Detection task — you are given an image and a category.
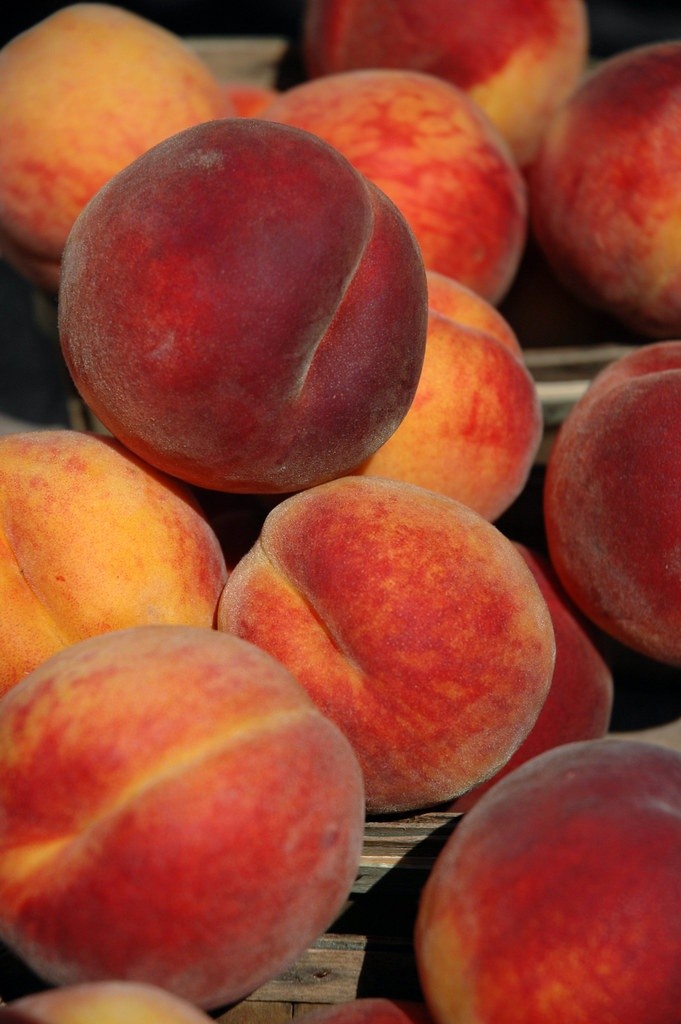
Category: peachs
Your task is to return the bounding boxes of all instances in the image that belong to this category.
[1,1,681,1024]
